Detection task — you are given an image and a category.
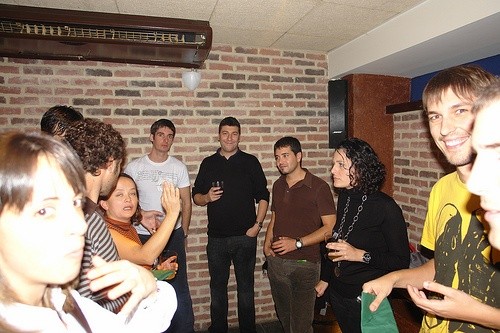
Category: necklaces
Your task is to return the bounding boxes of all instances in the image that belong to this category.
[334,194,368,267]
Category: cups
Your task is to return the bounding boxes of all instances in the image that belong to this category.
[158,250,178,280]
[212,180,223,195]
[324,230,344,261]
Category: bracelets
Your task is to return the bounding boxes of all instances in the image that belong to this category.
[183,235,189,239]
[255,220,263,226]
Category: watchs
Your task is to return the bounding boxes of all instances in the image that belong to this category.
[363,250,371,264]
[294,236,304,250]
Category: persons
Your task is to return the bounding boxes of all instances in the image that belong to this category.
[313,137,411,333]
[98,172,182,280]
[467,75,500,250]
[361,64,500,333]
[192,117,270,333]
[123,118,196,333]
[262,136,337,333]
[0,104,177,333]
